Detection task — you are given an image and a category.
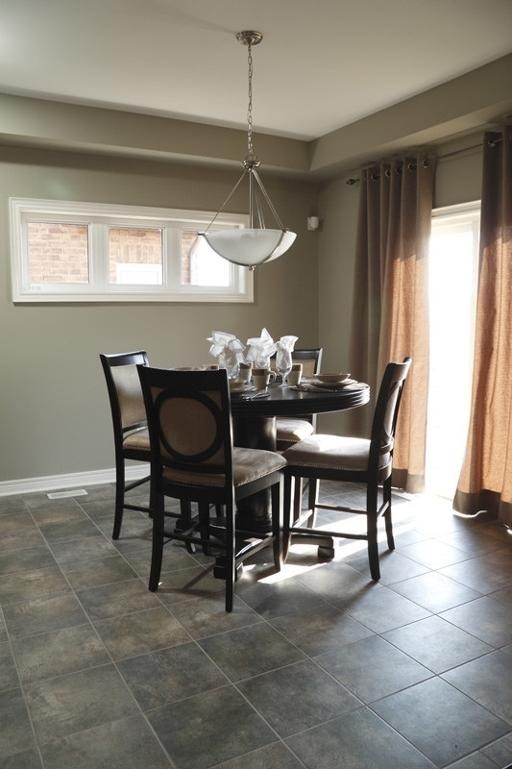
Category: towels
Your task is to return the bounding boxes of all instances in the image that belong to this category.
[273,335,299,369]
[206,330,247,366]
[246,328,279,370]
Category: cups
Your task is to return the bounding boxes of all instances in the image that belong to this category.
[240,362,252,384]
[210,365,219,370]
[252,368,277,389]
[288,363,303,386]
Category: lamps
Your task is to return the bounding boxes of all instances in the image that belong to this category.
[197,30,297,271]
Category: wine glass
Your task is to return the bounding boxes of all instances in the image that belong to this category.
[275,350,292,387]
[254,355,271,371]
[220,350,239,379]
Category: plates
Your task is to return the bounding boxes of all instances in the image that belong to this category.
[315,373,351,384]
[311,381,356,390]
